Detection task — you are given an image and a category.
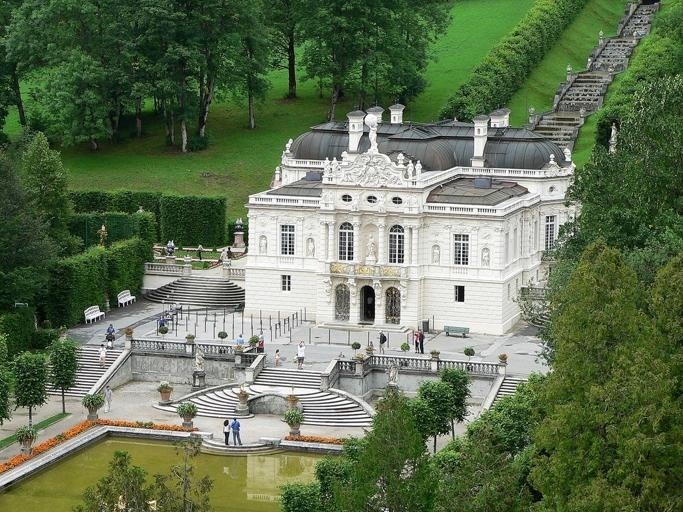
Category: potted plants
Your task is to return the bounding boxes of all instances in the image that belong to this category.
[16,425,37,448]
[499,353,508,363]
[60,325,67,337]
[430,351,440,358]
[82,394,105,420]
[248,335,259,347]
[281,408,304,436]
[185,335,195,343]
[218,331,228,354]
[464,348,475,371]
[177,402,197,428]
[158,384,173,401]
[401,343,410,366]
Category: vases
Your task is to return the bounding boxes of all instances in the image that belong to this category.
[238,393,249,404]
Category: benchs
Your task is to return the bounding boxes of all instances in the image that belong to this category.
[117,290,136,307]
[442,326,469,338]
[84,306,105,324]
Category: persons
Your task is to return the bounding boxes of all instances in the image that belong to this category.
[331,156,339,174]
[418,328,425,355]
[97,344,107,369]
[296,339,305,369]
[376,330,387,354]
[406,159,414,181]
[293,353,298,362]
[367,123,379,153]
[222,419,230,446]
[259,237,266,253]
[234,333,245,345]
[257,329,264,340]
[225,246,232,260]
[157,314,167,328]
[194,346,205,371]
[482,248,489,267]
[366,234,377,258]
[432,246,439,264]
[307,239,314,256]
[215,248,227,265]
[102,385,113,413]
[104,323,115,342]
[272,349,280,367]
[414,159,422,179]
[230,417,242,446]
[414,331,420,355]
[239,246,248,258]
[195,242,203,260]
[104,330,115,349]
[322,156,331,177]
